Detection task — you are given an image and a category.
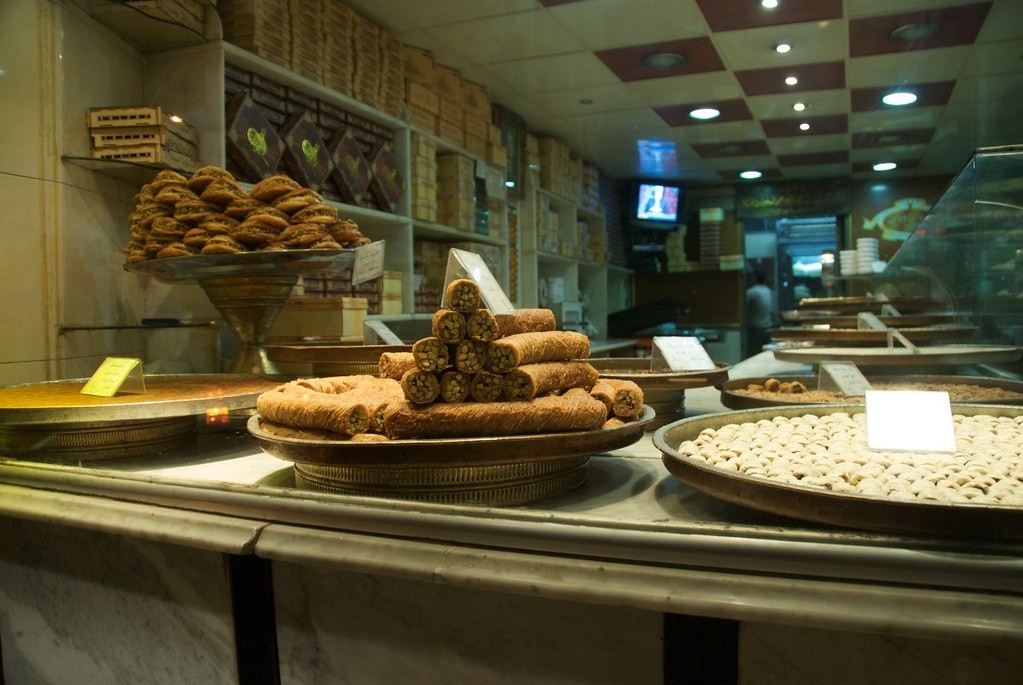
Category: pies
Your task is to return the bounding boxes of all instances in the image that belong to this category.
[124,167,371,263]
[257,279,642,443]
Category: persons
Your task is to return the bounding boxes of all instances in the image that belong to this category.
[646,186,668,214]
[744,267,773,356]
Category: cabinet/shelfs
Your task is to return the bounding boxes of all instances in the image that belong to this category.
[516,178,608,360]
[57,0,508,382]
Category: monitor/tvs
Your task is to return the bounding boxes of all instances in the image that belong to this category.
[625,177,686,231]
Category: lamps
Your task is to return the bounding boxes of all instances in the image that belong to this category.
[687,103,725,122]
[641,51,685,70]
[881,87,922,106]
[889,20,940,43]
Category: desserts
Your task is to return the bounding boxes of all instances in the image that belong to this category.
[678,379,1023,506]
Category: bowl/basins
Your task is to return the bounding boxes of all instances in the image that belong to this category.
[839,238,879,275]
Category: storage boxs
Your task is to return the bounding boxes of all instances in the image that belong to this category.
[698,208,746,273]
[83,0,608,348]
